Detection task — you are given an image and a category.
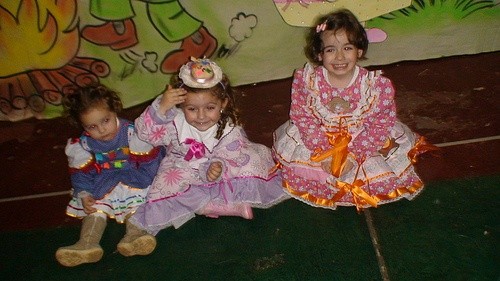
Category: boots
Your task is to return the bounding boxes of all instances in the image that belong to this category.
[117,214,156,257]
[203,204,253,219]
[55,216,107,267]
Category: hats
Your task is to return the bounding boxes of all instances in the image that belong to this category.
[180,56,222,88]
